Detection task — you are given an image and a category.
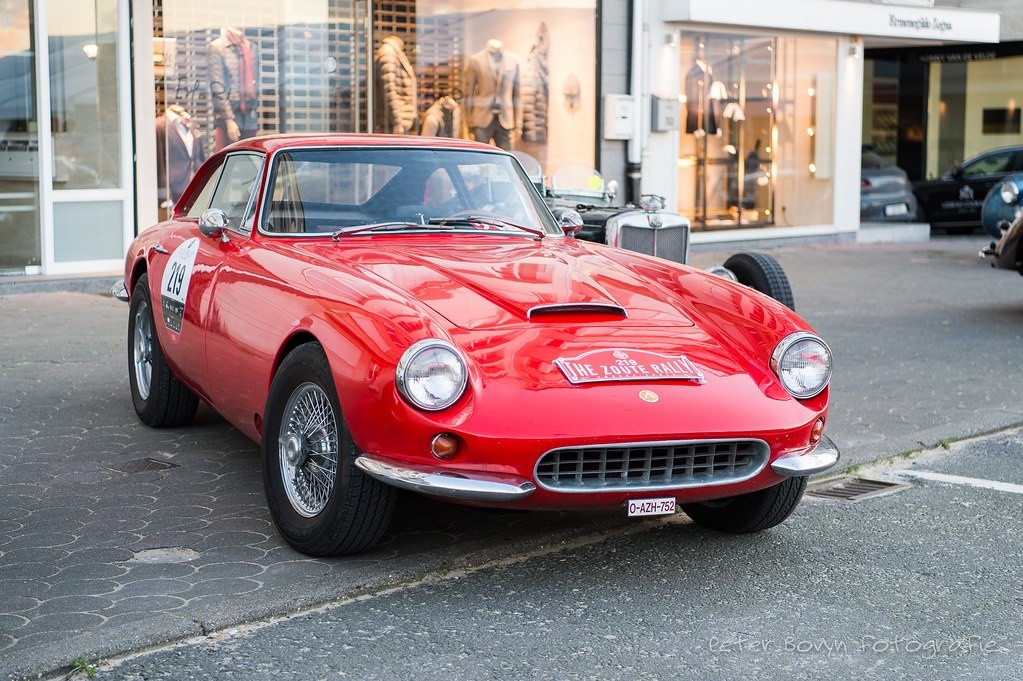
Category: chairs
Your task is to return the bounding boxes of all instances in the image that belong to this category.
[394,206,444,226]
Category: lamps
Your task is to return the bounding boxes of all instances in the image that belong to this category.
[82,44,98,59]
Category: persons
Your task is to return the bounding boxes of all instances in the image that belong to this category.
[206,25,258,153]
[155,104,205,204]
[464,39,521,152]
[520,52,548,144]
[683,59,725,136]
[419,96,458,138]
[373,33,418,135]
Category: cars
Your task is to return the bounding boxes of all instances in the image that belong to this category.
[861,145,1023,237]
[108,133,833,558]
